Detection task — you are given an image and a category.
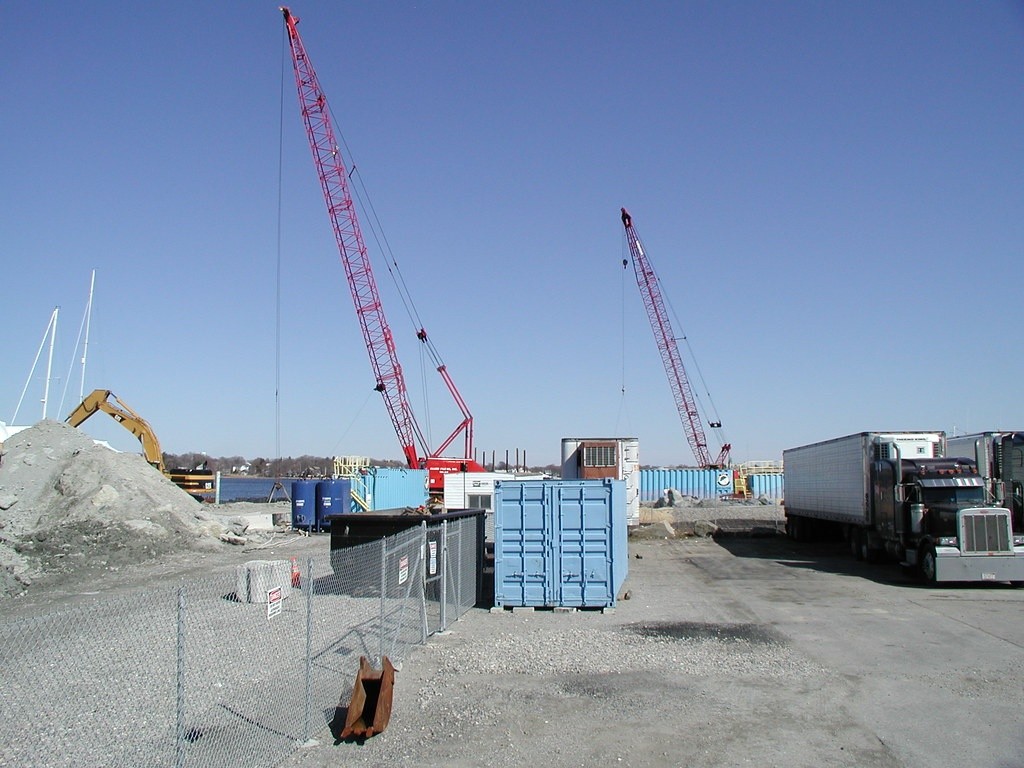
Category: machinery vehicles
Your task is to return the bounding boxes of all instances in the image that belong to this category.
[65,388,217,501]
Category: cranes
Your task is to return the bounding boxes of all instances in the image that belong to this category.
[619,204,731,472]
[276,4,488,497]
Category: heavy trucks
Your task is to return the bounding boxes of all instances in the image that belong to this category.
[781,430,993,582]
